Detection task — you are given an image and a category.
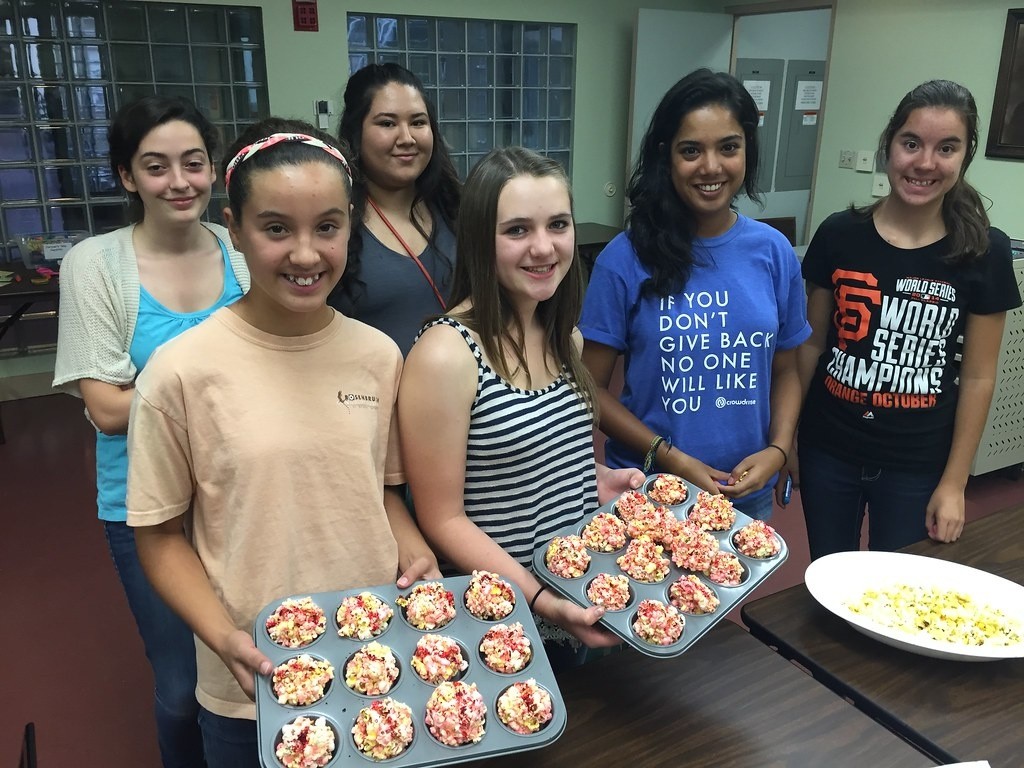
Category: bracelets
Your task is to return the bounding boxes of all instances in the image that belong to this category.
[768,444,788,467]
[644,435,673,476]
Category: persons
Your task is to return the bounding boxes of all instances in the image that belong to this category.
[398,145,645,671]
[58,93,252,768]
[125,117,446,768]
[770,79,1023,563]
[326,63,471,361]
[579,68,814,528]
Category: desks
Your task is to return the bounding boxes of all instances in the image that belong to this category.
[575,222,628,285]
[432,617,937,767]
[740,508,1024,768]
[0,262,58,336]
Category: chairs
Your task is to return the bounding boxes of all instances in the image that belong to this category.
[755,216,796,248]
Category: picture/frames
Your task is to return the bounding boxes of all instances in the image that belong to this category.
[985,8,1024,162]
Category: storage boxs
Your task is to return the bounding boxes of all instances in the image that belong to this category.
[15,232,91,271]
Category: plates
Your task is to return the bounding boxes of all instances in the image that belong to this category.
[804,551,1024,660]
